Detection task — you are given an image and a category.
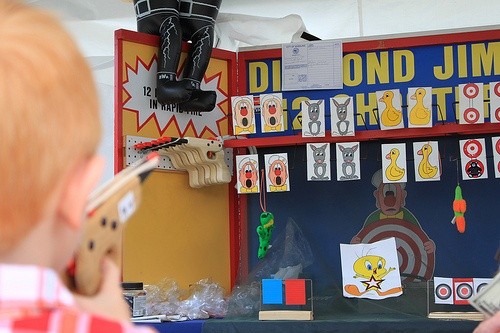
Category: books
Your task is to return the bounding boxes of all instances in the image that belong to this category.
[259,310,313,320]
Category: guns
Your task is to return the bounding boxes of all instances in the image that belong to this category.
[132,134,232,191]
[62,151,163,298]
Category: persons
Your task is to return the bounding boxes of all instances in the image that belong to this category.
[473,311,500,333]
[0,0,157,333]
